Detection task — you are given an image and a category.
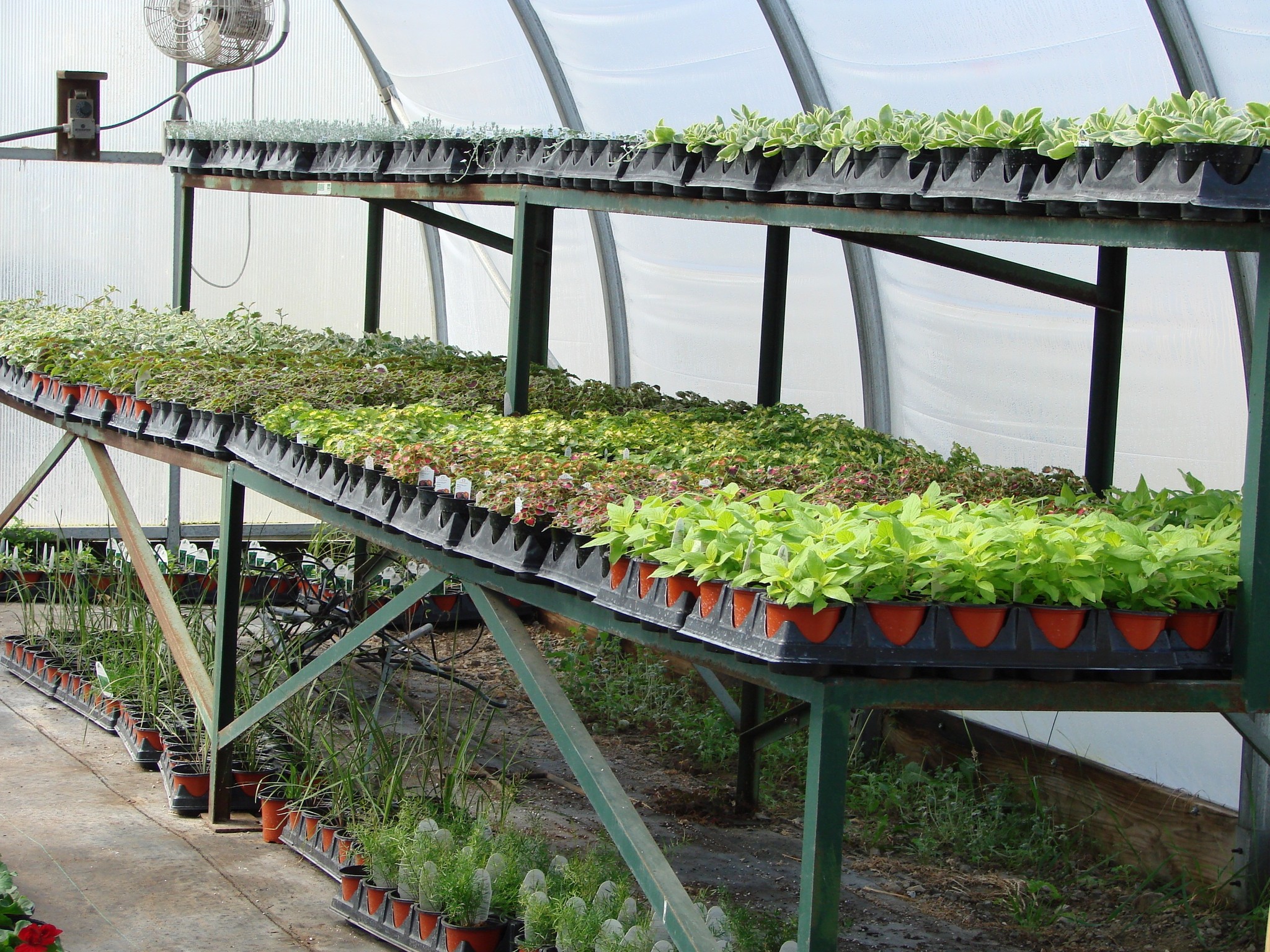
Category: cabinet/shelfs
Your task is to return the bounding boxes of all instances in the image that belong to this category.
[0,170,1270,952]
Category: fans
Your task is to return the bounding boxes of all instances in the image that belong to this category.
[142,0,276,70]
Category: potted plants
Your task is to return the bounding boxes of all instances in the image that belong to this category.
[0,98,1270,952]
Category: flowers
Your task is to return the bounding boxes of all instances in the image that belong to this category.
[0,861,65,952]
